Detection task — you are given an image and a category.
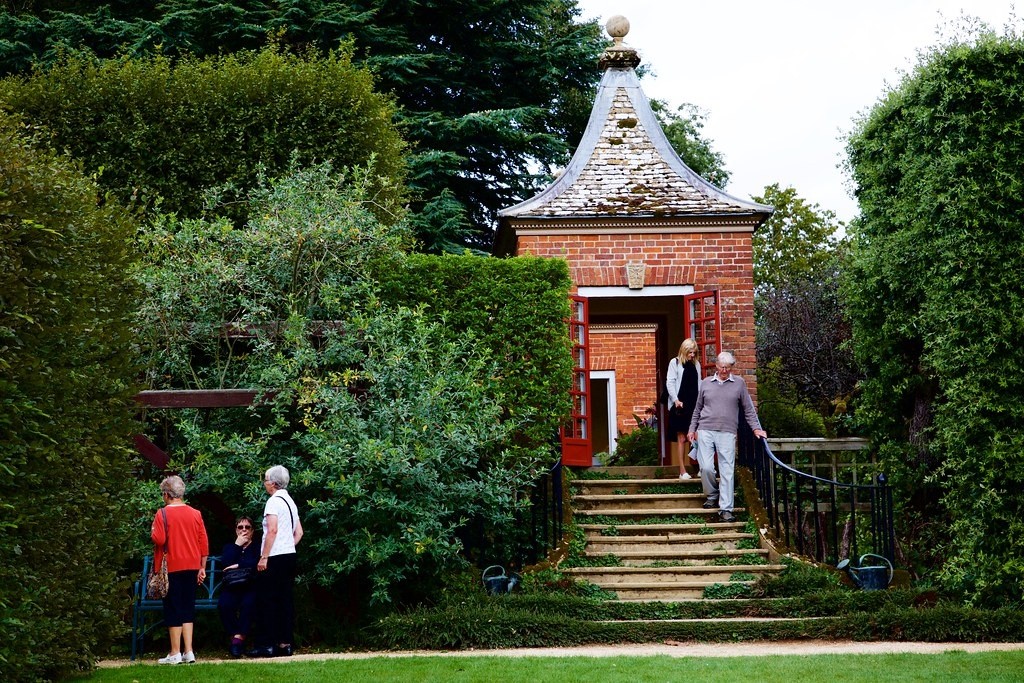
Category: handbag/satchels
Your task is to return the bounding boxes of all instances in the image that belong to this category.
[147,553,169,600]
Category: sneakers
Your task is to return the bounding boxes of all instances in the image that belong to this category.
[182,650,196,663]
[158,651,182,664]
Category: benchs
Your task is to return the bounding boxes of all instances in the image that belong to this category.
[131,556,222,660]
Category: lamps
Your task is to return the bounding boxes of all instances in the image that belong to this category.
[625,261,647,290]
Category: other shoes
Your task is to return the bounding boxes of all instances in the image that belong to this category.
[242,645,274,657]
[717,510,735,522]
[228,644,241,657]
[679,472,692,479]
[697,471,702,478]
[703,498,719,508]
[276,643,293,655]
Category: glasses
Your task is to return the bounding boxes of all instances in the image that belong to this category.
[236,525,252,530]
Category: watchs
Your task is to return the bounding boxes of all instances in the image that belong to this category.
[261,556,268,559]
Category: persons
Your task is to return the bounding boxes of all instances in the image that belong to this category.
[687,351,767,521]
[666,338,702,480]
[150,475,209,665]
[218,515,260,656]
[253,465,303,657]
